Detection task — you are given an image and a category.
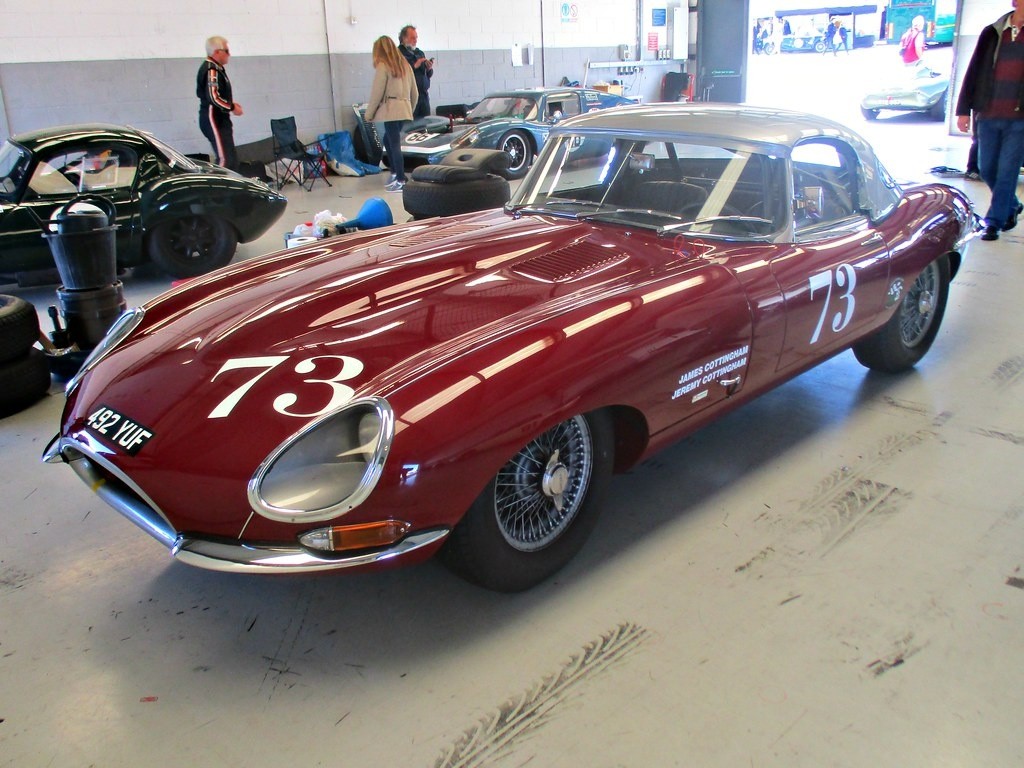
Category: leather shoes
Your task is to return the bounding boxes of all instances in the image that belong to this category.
[981,225,1000,241]
[1001,200,1023,231]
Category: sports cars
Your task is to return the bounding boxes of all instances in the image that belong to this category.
[860,62,951,121]
[377,86,654,182]
[39,101,989,595]
[0,123,288,279]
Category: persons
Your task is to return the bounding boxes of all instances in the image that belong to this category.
[196,36,243,174]
[821,18,838,56]
[398,26,433,117]
[365,36,419,192]
[835,23,849,55]
[964,109,984,181]
[752,18,791,55]
[900,15,924,67]
[955,0,1024,240]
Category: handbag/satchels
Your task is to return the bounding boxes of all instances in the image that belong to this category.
[899,48,906,55]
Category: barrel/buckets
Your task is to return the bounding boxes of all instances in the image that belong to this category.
[41,224,119,290]
[55,279,127,350]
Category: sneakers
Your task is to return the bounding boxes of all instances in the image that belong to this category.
[384,174,398,188]
[386,181,405,193]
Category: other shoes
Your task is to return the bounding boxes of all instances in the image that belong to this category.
[965,172,984,181]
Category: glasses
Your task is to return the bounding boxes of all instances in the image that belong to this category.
[218,49,229,54]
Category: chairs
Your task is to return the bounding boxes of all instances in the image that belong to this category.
[271,116,333,191]
[631,180,707,215]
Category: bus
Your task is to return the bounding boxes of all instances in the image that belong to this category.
[755,3,878,55]
[883,0,958,46]
